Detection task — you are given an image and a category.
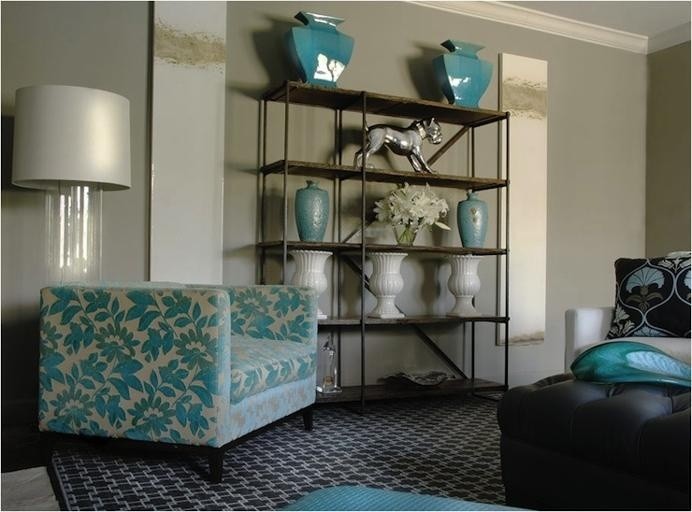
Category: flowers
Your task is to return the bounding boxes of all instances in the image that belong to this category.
[371,181,451,244]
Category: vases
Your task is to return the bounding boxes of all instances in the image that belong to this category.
[363,252,404,318]
[294,178,329,244]
[456,191,489,249]
[433,39,495,108]
[288,250,333,318]
[388,216,422,247]
[286,11,356,87]
[443,253,484,318]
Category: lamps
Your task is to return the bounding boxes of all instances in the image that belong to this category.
[11,85,131,282]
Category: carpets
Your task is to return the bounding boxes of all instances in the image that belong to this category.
[49,388,510,511]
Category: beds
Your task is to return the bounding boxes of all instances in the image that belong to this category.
[496,250,690,511]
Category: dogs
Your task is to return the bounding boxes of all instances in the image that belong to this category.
[354,115,443,174]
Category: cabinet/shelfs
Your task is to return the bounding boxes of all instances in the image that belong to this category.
[259,80,511,419]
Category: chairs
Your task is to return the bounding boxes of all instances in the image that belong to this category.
[34,282,318,486]
